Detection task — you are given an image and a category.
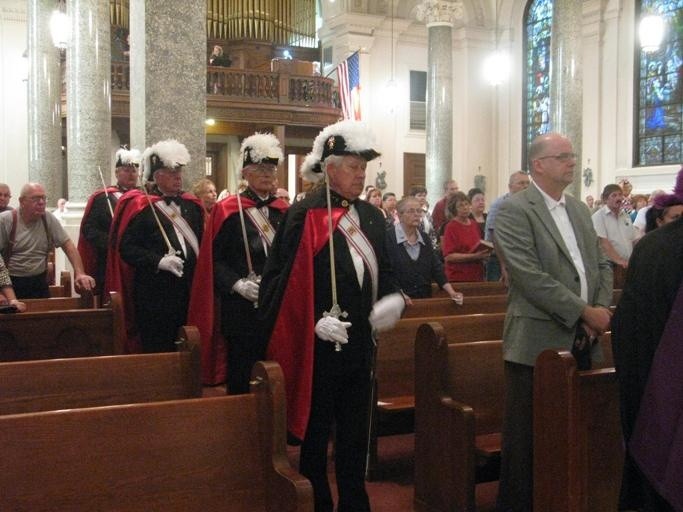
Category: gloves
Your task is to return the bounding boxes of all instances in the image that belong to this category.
[232,279,260,302]
[368,293,406,332]
[158,256,185,278]
[315,316,352,345]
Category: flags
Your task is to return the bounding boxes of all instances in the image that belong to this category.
[334,51,365,119]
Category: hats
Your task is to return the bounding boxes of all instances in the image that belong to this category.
[299,119,383,184]
[142,139,191,181]
[115,144,142,167]
[240,130,285,179]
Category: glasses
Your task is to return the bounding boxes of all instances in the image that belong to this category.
[539,152,579,160]
[22,194,47,201]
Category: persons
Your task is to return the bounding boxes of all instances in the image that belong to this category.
[0,186,12,212]
[0,183,96,313]
[123,35,130,62]
[207,45,223,93]
[312,60,322,77]
[489,135,616,511]
[615,217,683,508]
[75,150,142,295]
[185,133,291,397]
[51,197,68,215]
[116,139,205,353]
[192,168,682,308]
[247,119,410,509]
